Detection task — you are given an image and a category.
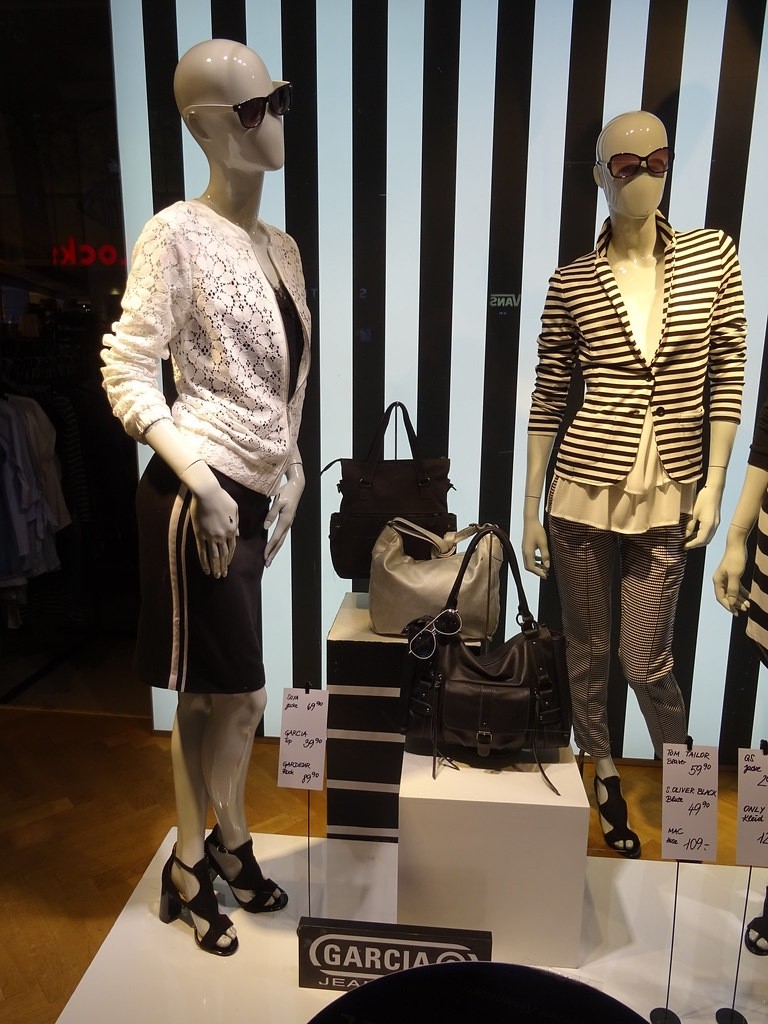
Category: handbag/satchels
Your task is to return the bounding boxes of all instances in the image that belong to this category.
[369,517,503,641]
[400,526,573,797]
[320,401,457,578]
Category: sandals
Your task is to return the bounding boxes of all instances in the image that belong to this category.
[594,771,642,859]
[744,887,768,956]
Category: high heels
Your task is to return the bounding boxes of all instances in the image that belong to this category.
[159,842,238,957]
[205,823,288,912]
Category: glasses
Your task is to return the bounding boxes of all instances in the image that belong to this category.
[409,609,462,661]
[595,147,675,179]
[184,80,292,128]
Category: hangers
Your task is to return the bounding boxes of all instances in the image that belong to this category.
[0,355,60,399]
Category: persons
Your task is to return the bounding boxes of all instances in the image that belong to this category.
[101,37,311,957]
[713,314,768,959]
[521,111,747,861]
